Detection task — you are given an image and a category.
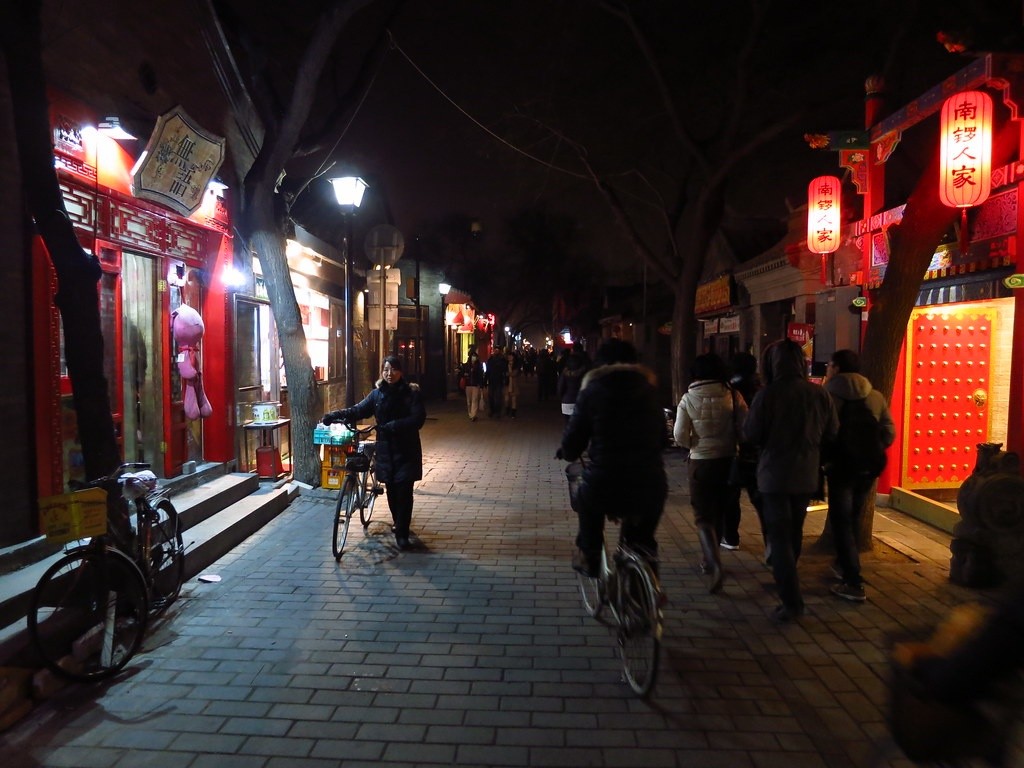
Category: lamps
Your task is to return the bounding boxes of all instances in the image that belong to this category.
[94,115,138,140]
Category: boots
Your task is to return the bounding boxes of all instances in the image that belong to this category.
[505,406,511,415]
[697,526,725,594]
[512,408,517,418]
[698,529,722,574]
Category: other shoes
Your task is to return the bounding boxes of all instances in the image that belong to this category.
[391,523,396,533]
[396,536,411,551]
[761,580,779,594]
[774,604,804,622]
[571,556,601,577]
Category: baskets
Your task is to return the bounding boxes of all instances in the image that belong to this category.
[313,427,355,445]
[331,435,379,473]
[565,460,591,513]
[38,484,108,546]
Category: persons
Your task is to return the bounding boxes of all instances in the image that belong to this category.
[674,353,749,595]
[720,352,772,565]
[556,337,676,606]
[742,338,840,622]
[459,342,592,422]
[322,358,427,549]
[822,349,894,601]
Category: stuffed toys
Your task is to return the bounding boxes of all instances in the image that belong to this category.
[170,303,213,421]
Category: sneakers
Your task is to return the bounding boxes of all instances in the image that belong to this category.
[720,537,739,550]
[832,584,866,601]
[829,559,844,580]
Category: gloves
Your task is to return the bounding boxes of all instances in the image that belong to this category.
[381,420,400,435]
[323,413,337,426]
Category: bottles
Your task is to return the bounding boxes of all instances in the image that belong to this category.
[327,446,353,478]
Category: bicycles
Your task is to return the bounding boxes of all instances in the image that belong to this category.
[322,417,390,559]
[552,451,666,704]
[28,462,196,688]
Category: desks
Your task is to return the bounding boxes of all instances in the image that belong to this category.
[243,418,293,482]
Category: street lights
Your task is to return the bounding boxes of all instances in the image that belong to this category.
[326,174,371,452]
[437,278,451,401]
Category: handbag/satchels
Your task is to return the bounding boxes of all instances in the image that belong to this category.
[479,391,486,412]
[457,376,466,389]
[732,390,753,489]
[501,370,509,385]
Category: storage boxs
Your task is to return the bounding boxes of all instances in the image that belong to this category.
[313,427,354,446]
[320,442,353,468]
[322,468,349,489]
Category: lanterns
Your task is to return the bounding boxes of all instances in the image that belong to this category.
[939,91,992,251]
[807,175,841,282]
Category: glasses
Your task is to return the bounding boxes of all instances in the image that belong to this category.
[824,361,832,366]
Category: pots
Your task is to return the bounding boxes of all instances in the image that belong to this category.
[250,401,282,424]
